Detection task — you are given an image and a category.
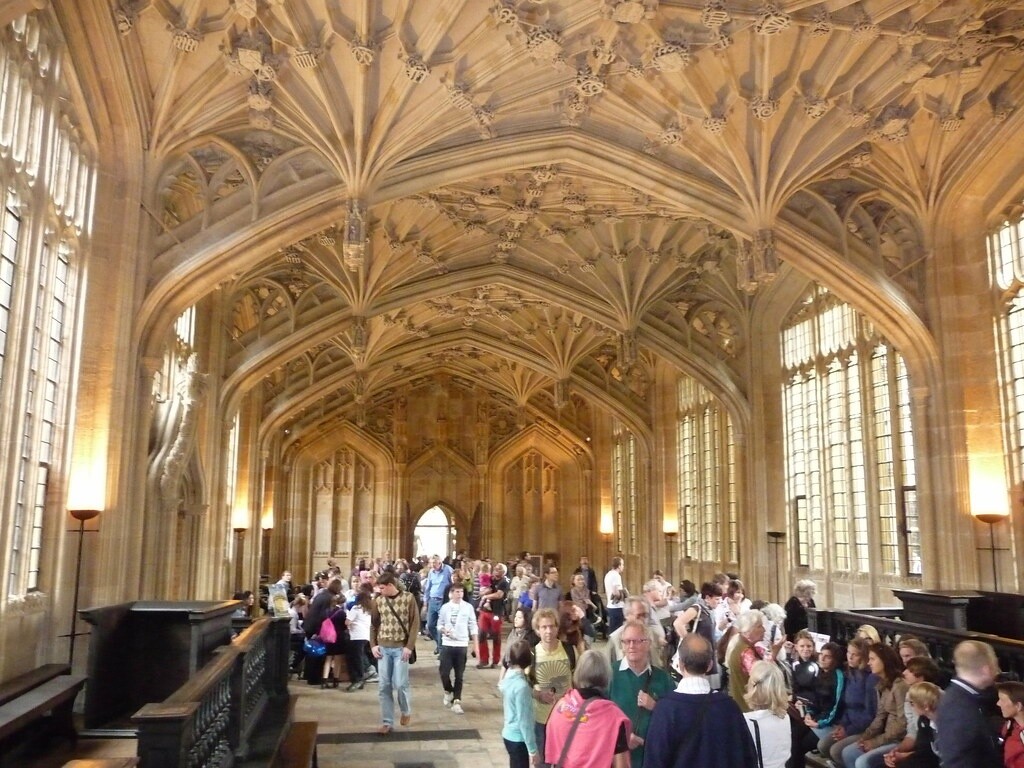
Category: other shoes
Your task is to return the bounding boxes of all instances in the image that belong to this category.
[382,724,392,735]
[425,636,432,641]
[400,714,410,724]
[483,603,493,612]
[477,607,483,612]
[365,672,379,682]
[491,663,497,669]
[476,661,489,668]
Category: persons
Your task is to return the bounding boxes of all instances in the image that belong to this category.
[642,634,758,768]
[608,620,677,768]
[742,661,806,768]
[370,574,420,734]
[523,607,579,768]
[785,579,1024,768]
[436,583,480,714]
[545,651,631,768]
[230,550,786,711]
[497,640,541,768]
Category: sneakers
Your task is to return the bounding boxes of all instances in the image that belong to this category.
[443,691,453,705]
[451,703,464,714]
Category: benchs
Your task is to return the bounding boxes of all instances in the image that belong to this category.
[0,675,89,768]
[0,664,75,725]
[267,722,318,768]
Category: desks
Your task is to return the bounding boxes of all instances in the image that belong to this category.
[77,599,242,733]
[895,589,991,633]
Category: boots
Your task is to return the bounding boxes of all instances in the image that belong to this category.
[333,678,339,687]
[321,678,331,688]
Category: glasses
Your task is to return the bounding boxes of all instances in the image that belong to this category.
[621,638,647,645]
[629,612,649,618]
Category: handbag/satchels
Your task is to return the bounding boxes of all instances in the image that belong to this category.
[404,634,417,664]
[321,619,337,643]
